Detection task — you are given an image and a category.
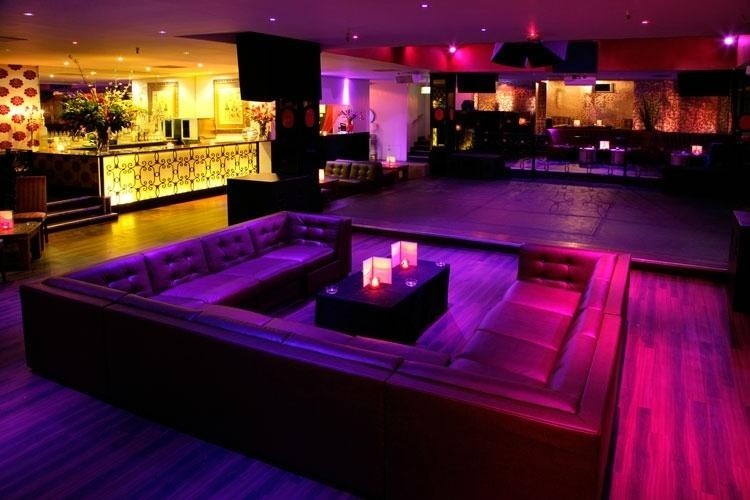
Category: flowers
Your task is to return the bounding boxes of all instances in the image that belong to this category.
[247,103,275,123]
[54,54,153,144]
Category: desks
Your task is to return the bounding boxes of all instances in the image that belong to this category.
[314,250,454,346]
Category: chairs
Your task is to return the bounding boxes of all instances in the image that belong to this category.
[12,175,49,250]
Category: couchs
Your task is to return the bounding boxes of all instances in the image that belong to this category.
[324,159,394,193]
[548,125,648,165]
[23,210,631,500]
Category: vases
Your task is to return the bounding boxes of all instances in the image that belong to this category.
[95,126,108,153]
[259,122,267,139]
